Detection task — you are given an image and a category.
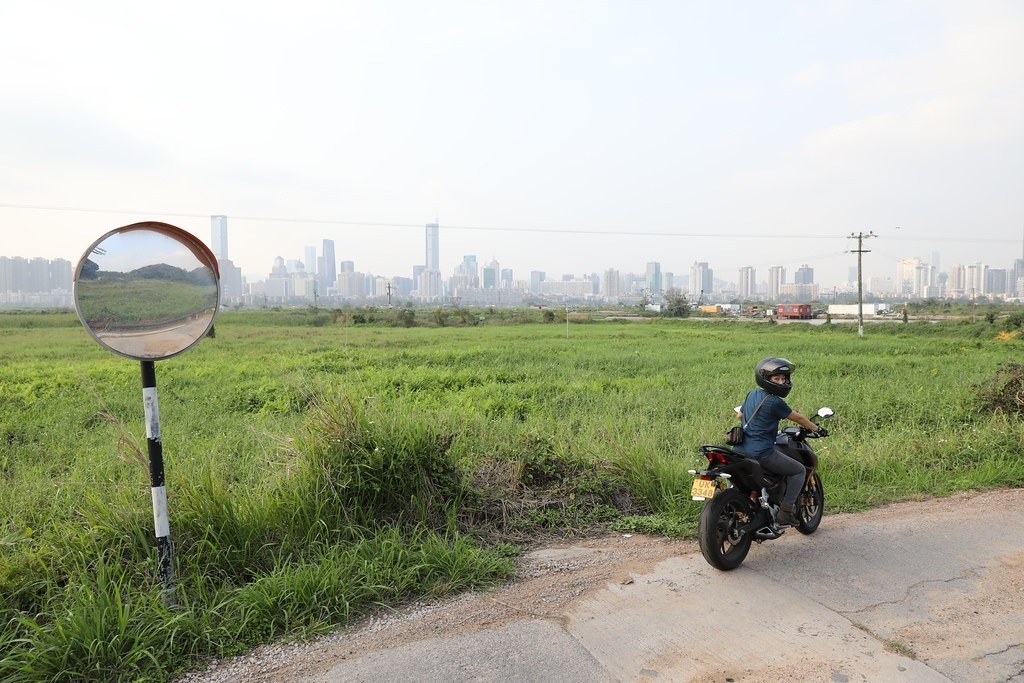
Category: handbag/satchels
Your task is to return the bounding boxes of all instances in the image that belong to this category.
[726,427,744,445]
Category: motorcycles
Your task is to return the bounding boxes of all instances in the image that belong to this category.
[690,407,835,572]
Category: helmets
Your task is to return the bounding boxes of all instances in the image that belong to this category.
[755,357,793,398]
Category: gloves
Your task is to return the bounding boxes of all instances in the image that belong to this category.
[813,426,827,437]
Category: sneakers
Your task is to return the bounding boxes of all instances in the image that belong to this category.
[777,510,800,526]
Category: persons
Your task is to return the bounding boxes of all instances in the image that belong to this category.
[731,356,828,527]
